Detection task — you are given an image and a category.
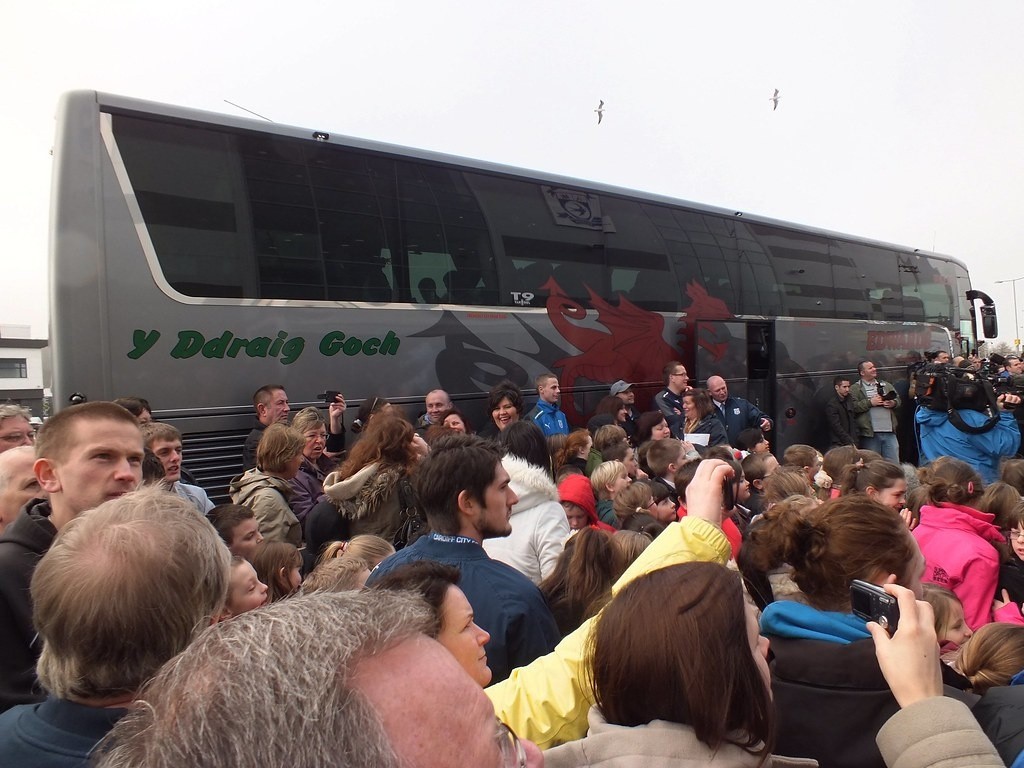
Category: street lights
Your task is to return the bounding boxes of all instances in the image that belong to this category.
[995,276,1024,355]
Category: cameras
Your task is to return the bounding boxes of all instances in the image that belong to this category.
[876,387,898,408]
[720,475,735,510]
[849,578,900,634]
[317,390,341,402]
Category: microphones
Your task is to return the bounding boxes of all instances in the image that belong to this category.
[1006,373,1023,387]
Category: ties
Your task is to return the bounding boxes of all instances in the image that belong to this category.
[720,404,725,419]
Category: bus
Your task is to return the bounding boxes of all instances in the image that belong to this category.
[43,86,998,505]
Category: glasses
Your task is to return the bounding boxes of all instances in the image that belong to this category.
[496,717,527,768]
[667,372,688,379]
[645,498,658,509]
[1004,531,1024,540]
[303,431,330,442]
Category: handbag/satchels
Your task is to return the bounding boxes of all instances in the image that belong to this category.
[393,476,427,552]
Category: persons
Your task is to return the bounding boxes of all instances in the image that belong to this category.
[0,349,1024,768]
[418,276,439,303]
[443,262,736,313]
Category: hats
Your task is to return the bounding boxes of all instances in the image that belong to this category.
[959,360,974,369]
[610,380,636,397]
